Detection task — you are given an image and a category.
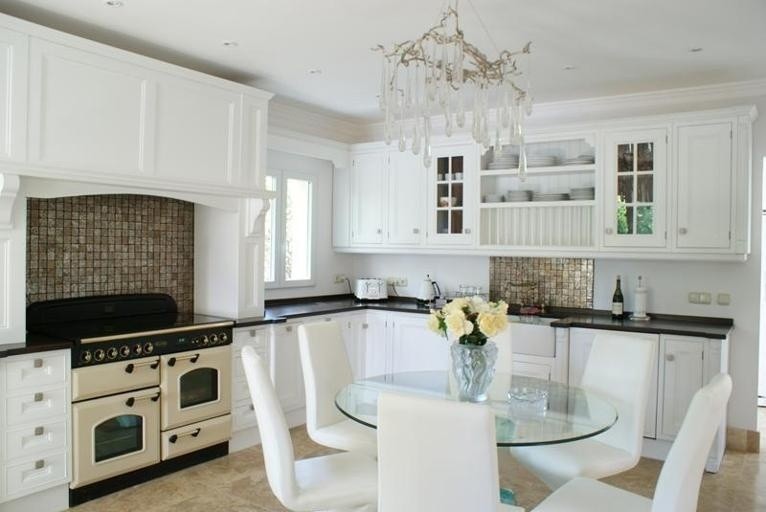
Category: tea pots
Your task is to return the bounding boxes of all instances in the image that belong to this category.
[416,274,442,304]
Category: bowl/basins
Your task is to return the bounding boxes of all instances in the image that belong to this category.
[439,197,458,208]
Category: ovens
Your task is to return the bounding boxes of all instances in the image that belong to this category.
[70,345,233,506]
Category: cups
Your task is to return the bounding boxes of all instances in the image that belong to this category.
[453,284,482,298]
[437,171,463,180]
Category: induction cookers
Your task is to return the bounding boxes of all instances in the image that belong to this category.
[33,311,234,368]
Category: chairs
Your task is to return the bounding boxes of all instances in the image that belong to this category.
[529,368,737,511]
[238,342,379,511]
[509,328,664,493]
[369,390,526,512]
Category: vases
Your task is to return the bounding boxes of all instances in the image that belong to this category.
[447,339,500,400]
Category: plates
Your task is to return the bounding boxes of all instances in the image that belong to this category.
[507,386,548,403]
[488,152,594,169]
[484,187,594,201]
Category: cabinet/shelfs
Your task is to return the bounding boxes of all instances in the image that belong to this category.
[671,104,760,263]
[330,133,432,253]
[568,312,662,460]
[480,122,600,257]
[229,296,446,454]
[0,343,71,511]
[430,132,485,256]
[601,110,671,260]
[653,316,738,474]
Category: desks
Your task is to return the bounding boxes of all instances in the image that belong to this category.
[332,363,619,507]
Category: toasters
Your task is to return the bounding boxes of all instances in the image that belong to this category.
[353,277,390,303]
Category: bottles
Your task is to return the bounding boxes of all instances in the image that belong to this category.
[611,275,623,321]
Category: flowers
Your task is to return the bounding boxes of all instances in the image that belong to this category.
[426,295,511,347]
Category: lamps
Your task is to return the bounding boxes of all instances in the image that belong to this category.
[369,0,542,185]
